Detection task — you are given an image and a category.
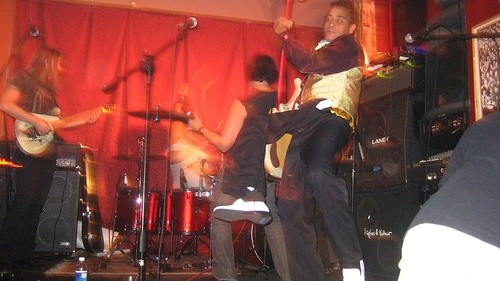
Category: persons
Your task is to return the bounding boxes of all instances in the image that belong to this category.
[0,45,102,269]
[398,110,500,281]
[186,54,291,281]
[165,124,214,187]
[212,0,365,281]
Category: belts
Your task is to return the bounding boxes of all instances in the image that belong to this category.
[329,107,355,133]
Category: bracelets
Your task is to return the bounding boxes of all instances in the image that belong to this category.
[198,125,205,134]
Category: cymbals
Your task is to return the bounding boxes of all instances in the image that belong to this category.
[113,151,167,163]
[126,108,192,123]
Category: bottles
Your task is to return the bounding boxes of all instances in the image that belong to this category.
[75,257,87,281]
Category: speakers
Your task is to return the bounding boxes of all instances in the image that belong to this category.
[348,185,425,281]
[425,0,471,116]
[350,91,424,190]
[31,141,81,252]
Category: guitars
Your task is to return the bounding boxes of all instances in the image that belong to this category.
[264,77,302,179]
[14,102,120,156]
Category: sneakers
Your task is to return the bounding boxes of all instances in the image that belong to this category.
[342,258,365,281]
[213,198,272,225]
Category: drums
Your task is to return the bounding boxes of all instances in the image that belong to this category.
[113,187,165,234]
[231,219,272,268]
[161,188,212,236]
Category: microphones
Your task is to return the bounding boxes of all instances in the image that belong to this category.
[405,23,439,43]
[28,24,39,37]
[176,17,197,29]
